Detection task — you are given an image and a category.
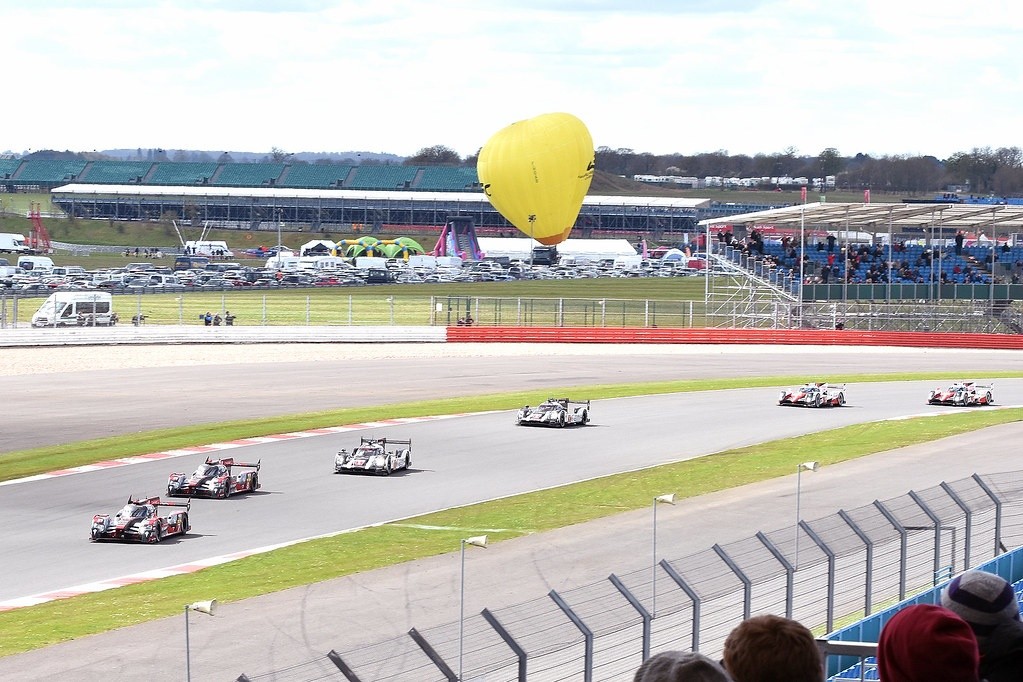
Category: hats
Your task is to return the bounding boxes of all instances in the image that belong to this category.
[633,650,734,682]
[940,569,1020,637]
[876,603,980,682]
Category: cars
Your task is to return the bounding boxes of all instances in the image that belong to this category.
[0,245,709,297]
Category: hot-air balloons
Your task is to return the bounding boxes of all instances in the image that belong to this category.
[476,112,596,268]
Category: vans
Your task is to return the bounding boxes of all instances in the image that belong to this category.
[31,291,112,329]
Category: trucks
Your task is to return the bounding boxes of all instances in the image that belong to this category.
[0,232,30,254]
[185,240,234,261]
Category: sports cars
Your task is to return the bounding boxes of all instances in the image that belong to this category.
[776,382,846,408]
[517,398,591,428]
[926,381,994,407]
[88,495,193,543]
[335,436,411,476]
[167,457,264,499]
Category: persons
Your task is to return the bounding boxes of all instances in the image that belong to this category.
[124,246,161,259]
[77,313,146,326]
[835,322,845,330]
[877,571,1023,682]
[715,230,1019,285]
[183,245,229,258]
[633,615,825,682]
[205,311,236,326]
